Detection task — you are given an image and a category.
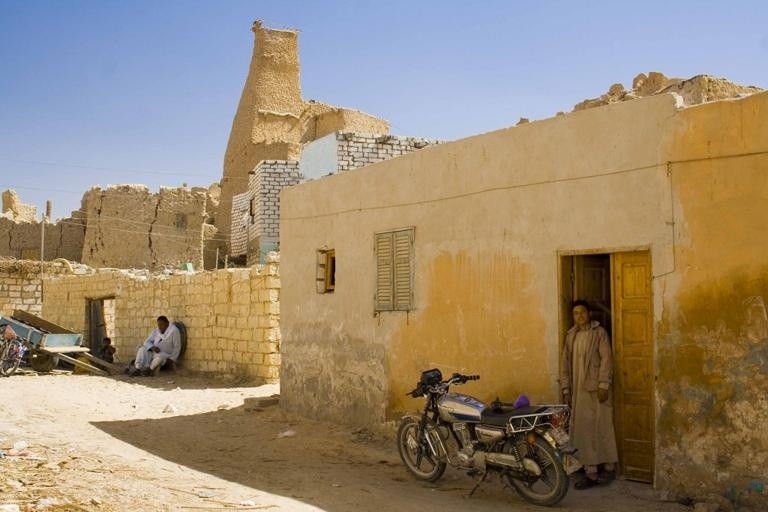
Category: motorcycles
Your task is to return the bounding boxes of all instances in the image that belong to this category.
[397,368,583,507]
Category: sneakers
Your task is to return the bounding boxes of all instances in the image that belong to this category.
[575,477,598,489]
[143,371,149,377]
[597,470,614,483]
[133,370,141,376]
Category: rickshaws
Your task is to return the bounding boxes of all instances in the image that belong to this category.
[0,310,125,375]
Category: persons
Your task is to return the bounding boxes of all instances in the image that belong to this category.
[129,315,181,377]
[98,338,115,371]
[561,300,620,491]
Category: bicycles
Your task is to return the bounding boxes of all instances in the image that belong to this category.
[0,328,26,376]
